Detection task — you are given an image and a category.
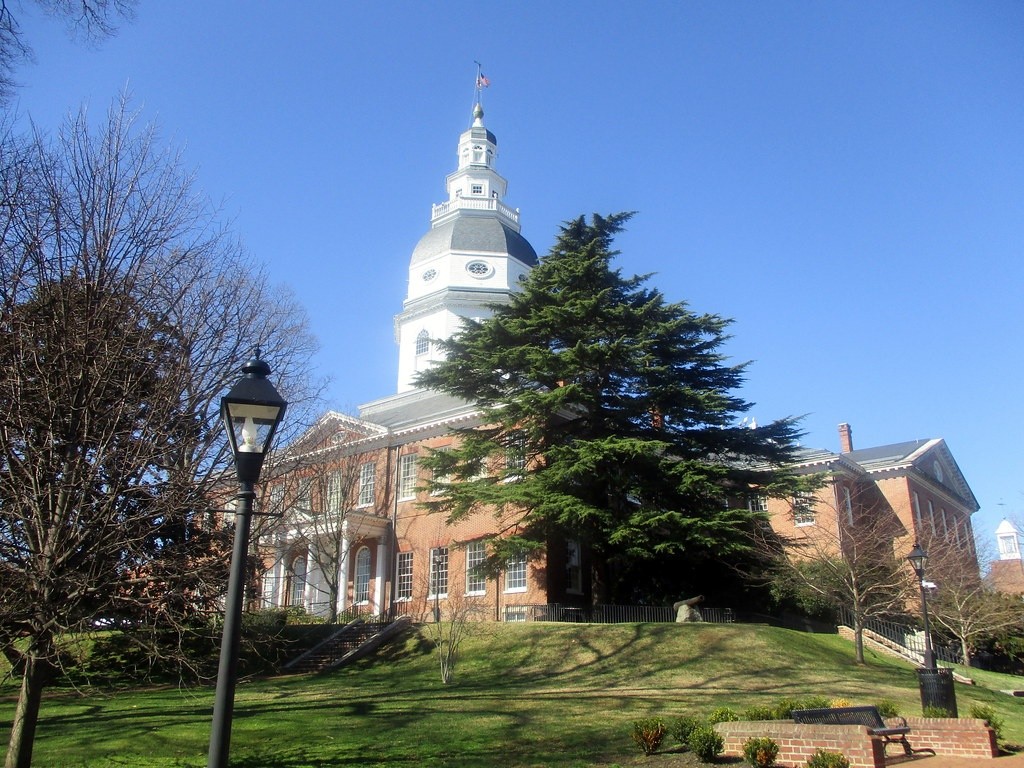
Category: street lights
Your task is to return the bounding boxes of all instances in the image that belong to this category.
[206,347,289,768]
[906,542,937,669]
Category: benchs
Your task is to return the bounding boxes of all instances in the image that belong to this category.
[790,705,911,759]
